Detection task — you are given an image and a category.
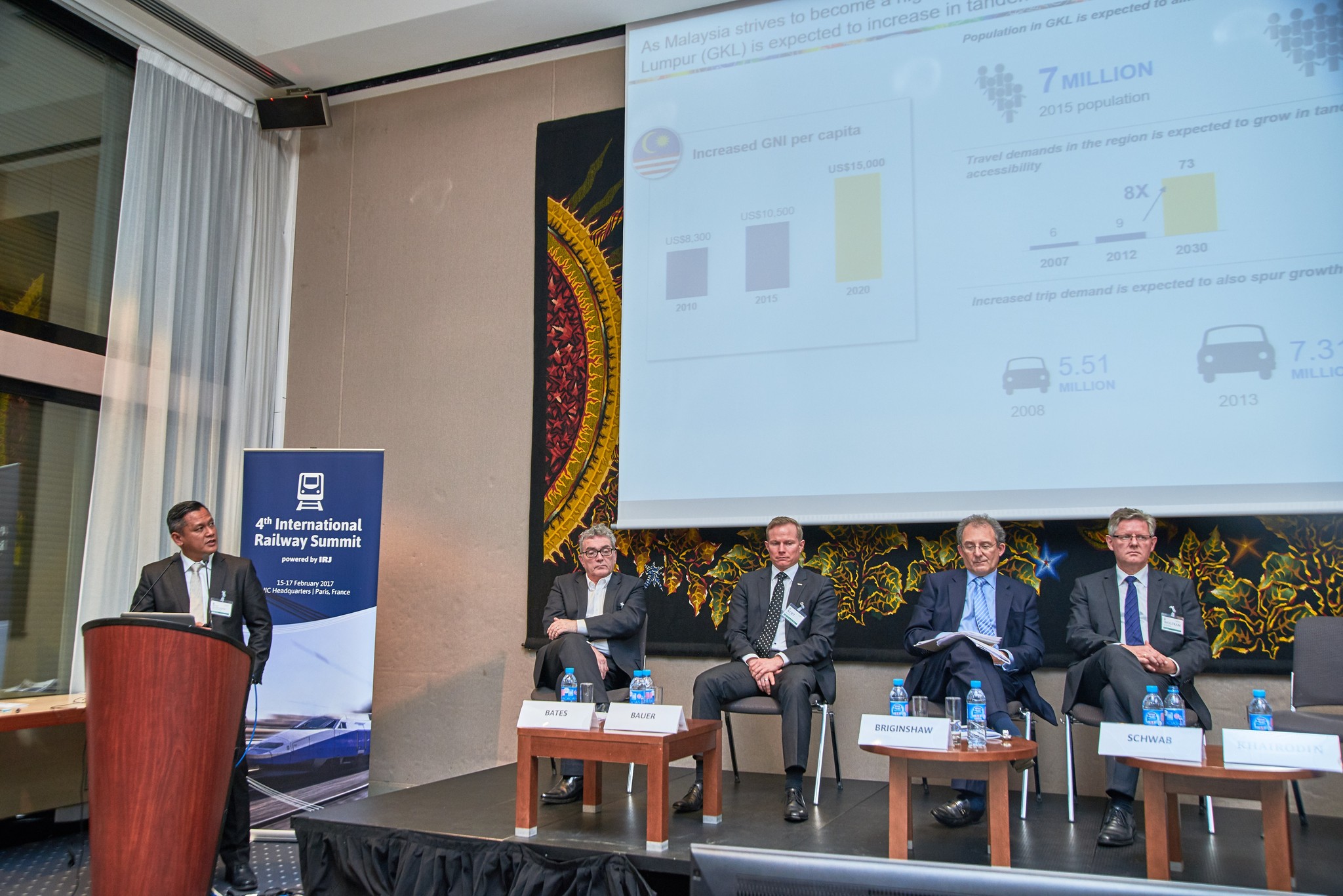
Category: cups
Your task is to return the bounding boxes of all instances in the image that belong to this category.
[579,683,594,703]
[945,697,961,745]
[912,696,929,717]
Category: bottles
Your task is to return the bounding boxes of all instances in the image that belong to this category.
[629,670,646,704]
[1247,689,1274,732]
[1163,685,1187,728]
[641,670,655,705]
[966,680,987,746]
[1142,685,1164,726]
[890,679,909,717]
[560,668,577,702]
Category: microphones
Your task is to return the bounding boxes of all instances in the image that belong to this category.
[130,551,180,612]
[202,553,213,630]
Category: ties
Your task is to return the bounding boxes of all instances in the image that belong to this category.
[755,572,788,659]
[1125,576,1144,647]
[189,563,204,627]
[971,577,1001,666]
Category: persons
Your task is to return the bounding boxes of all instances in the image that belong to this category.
[533,523,648,804]
[130,501,273,892]
[903,513,1058,828]
[1060,507,1213,847]
[672,516,836,823]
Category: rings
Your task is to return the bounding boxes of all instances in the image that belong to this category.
[1144,653,1146,657]
[556,627,558,630]
[764,678,769,681]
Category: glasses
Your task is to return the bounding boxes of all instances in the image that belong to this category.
[581,547,614,558]
[959,542,997,552]
[1111,534,1152,543]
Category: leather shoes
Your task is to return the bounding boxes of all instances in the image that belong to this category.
[1097,803,1138,845]
[595,702,610,713]
[225,859,257,890]
[541,775,582,803]
[782,787,808,821]
[930,793,986,826]
[1009,756,1036,773]
[672,781,704,811]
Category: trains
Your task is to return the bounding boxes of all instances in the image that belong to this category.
[245,712,371,775]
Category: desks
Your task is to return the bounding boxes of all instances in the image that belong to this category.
[860,734,1038,868]
[516,718,724,852]
[1114,744,1320,892]
[0,693,86,733]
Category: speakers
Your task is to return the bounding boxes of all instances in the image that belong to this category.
[254,92,333,131]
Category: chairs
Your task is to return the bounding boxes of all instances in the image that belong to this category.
[906,694,1044,820]
[721,699,844,804]
[530,613,649,794]
[1260,615,1343,840]
[1063,707,1216,835]
